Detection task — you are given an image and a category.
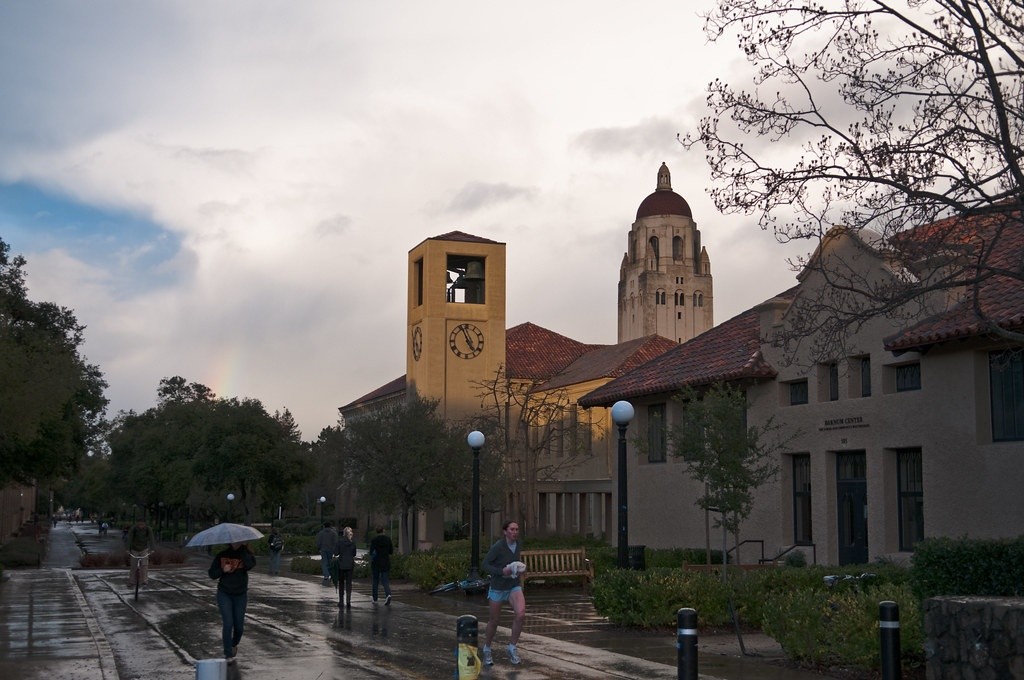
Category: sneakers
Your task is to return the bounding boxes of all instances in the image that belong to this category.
[506,645,521,664]
[483,649,493,665]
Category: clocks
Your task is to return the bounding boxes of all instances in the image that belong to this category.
[412,327,423,361]
[450,324,484,359]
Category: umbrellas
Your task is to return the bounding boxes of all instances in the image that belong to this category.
[185,522,265,564]
[326,555,339,594]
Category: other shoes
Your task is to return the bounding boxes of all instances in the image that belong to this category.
[384,595,391,605]
[372,600,378,604]
[226,646,238,663]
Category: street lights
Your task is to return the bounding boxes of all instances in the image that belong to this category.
[610,400,636,570]
[319,496,327,531]
[227,493,235,524]
[467,430,485,579]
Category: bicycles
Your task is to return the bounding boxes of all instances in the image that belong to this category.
[125,549,152,600]
[427,579,490,594]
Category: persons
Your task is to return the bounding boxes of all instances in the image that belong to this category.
[67,513,84,524]
[267,526,285,575]
[97,519,108,535]
[53,515,57,529]
[480,520,525,666]
[208,540,257,662]
[121,528,129,539]
[369,524,394,606]
[313,522,338,581]
[124,517,156,588]
[334,527,356,607]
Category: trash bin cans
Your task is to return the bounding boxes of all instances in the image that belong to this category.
[628,544,645,570]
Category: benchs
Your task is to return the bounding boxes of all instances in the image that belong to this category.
[520,546,594,593]
